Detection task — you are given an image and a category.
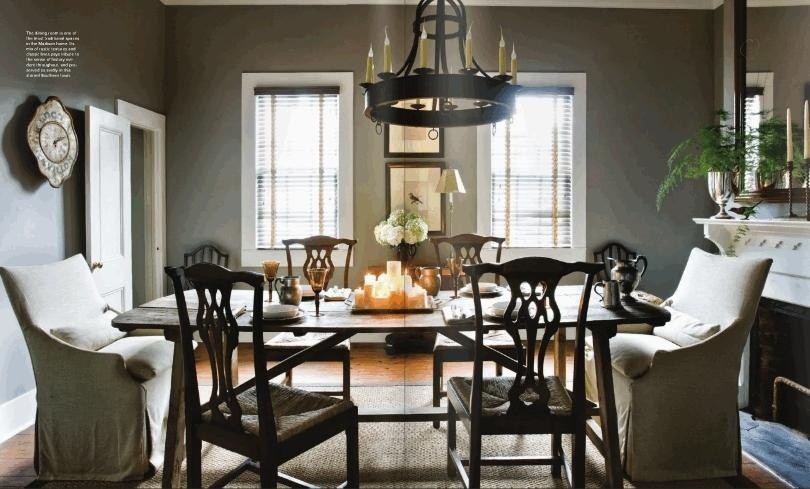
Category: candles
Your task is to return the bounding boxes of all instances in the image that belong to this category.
[354,261,427,310]
[786,106,793,161]
[803,99,809,156]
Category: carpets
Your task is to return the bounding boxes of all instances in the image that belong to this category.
[136,383,636,489]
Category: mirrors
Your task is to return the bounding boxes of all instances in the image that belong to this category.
[733,0,810,203]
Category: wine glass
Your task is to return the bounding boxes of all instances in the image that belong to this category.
[447,258,464,299]
[262,260,280,303]
[306,267,329,318]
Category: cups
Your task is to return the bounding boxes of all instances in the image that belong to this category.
[414,266,441,296]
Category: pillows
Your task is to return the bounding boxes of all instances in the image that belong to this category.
[653,303,720,349]
[49,315,126,350]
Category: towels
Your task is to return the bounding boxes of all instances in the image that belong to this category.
[441,305,475,323]
[324,286,351,301]
[506,283,540,295]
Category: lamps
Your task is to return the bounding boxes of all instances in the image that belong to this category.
[435,166,466,272]
[357,1,523,141]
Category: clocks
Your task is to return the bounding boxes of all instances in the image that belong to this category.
[25,96,82,187]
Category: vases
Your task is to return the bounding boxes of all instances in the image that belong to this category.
[392,243,417,261]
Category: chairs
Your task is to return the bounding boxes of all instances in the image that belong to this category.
[429,234,506,430]
[592,241,638,284]
[162,261,359,488]
[182,243,229,292]
[263,236,358,403]
[446,257,606,488]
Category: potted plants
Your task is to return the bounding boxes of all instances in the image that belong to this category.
[653,111,755,219]
[743,110,810,190]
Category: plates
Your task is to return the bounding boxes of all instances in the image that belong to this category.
[249,311,304,321]
[459,287,506,295]
[301,295,316,299]
[485,307,535,321]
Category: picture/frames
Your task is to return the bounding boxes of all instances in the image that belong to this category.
[383,96,446,156]
[385,162,446,235]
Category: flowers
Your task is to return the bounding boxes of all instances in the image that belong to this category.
[373,207,429,246]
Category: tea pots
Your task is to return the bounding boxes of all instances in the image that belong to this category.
[606,255,648,301]
[594,279,625,310]
[274,275,302,306]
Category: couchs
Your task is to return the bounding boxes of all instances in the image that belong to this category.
[579,247,773,483]
[0,251,200,486]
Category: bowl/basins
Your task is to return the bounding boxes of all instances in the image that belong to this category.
[298,284,314,296]
[262,304,298,318]
[466,282,498,292]
[492,301,521,316]
